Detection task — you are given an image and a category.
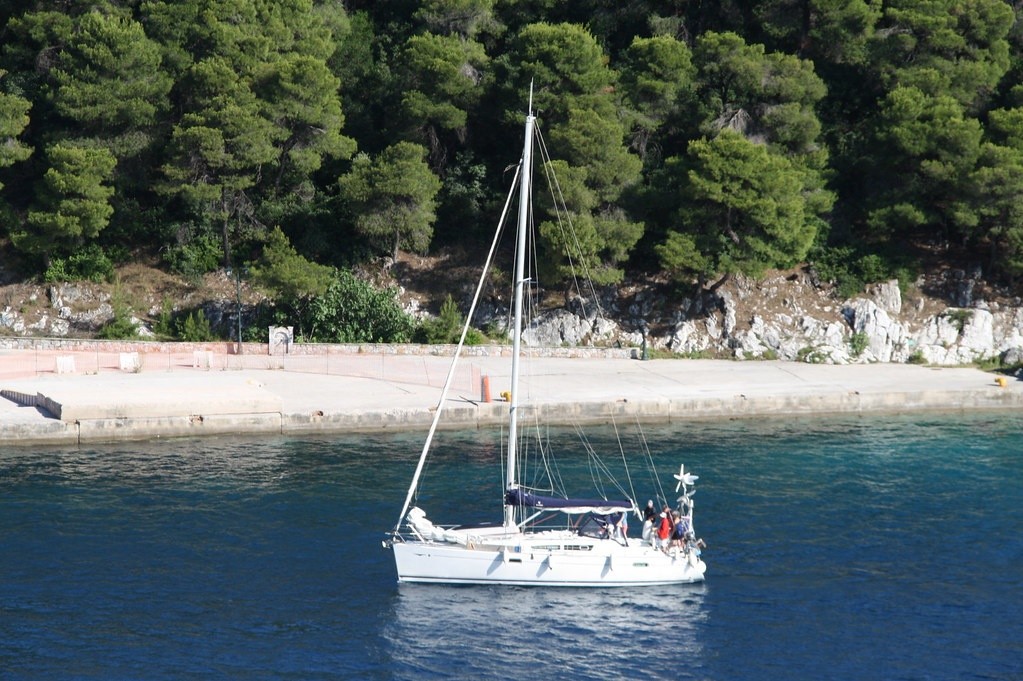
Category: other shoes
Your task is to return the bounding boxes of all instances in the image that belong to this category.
[626,541,630,547]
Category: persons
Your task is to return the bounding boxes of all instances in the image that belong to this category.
[617,511,629,547]
[642,501,684,548]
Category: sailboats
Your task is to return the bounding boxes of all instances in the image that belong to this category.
[381,74,708,590]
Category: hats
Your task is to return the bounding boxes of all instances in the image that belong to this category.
[660,512,667,519]
